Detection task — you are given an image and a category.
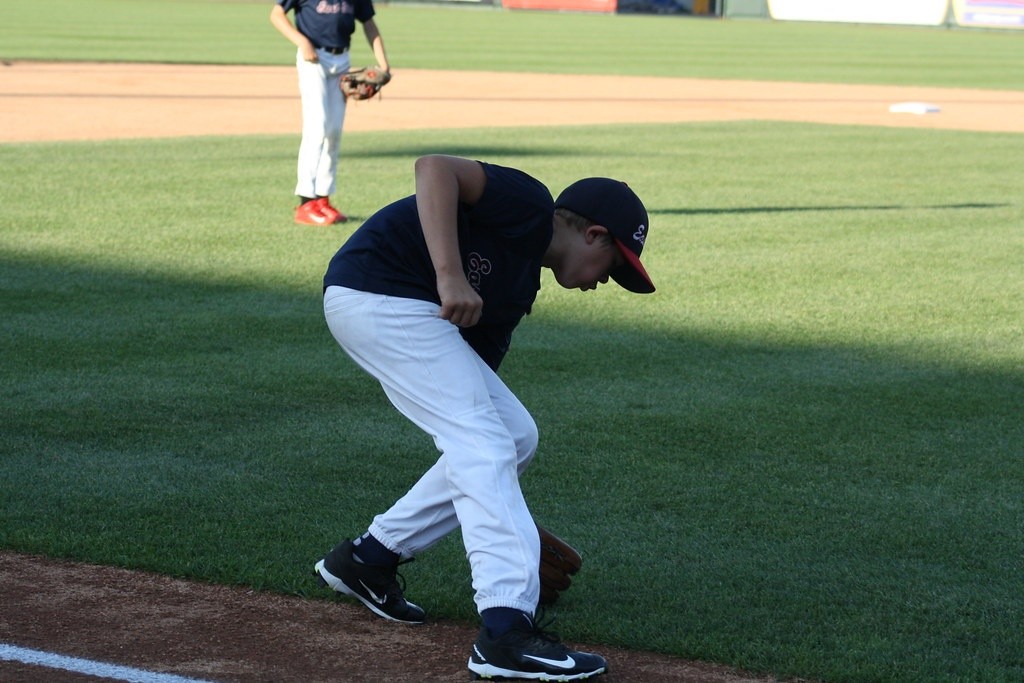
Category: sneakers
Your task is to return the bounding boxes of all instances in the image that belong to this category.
[468,607,608,683]
[317,198,347,224]
[295,199,334,226]
[312,539,425,624]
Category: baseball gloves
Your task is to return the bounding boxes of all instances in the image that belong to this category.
[532,519,585,606]
[339,65,392,104]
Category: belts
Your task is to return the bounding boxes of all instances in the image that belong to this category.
[314,43,344,54]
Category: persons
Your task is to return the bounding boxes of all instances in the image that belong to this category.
[312,156,655,683]
[271,0,388,225]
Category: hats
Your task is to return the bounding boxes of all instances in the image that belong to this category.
[555,177,656,294]
